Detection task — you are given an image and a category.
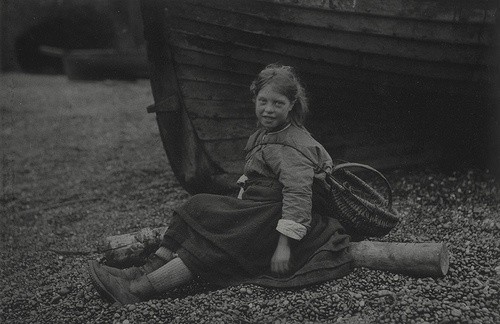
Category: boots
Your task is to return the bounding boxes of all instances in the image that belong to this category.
[102,266,141,280]
[88,259,156,304]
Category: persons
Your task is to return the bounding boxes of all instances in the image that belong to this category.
[88,64,333,307]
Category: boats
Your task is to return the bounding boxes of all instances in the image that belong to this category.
[136,0,500,196]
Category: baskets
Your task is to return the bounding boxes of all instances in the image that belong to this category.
[327,158,399,240]
